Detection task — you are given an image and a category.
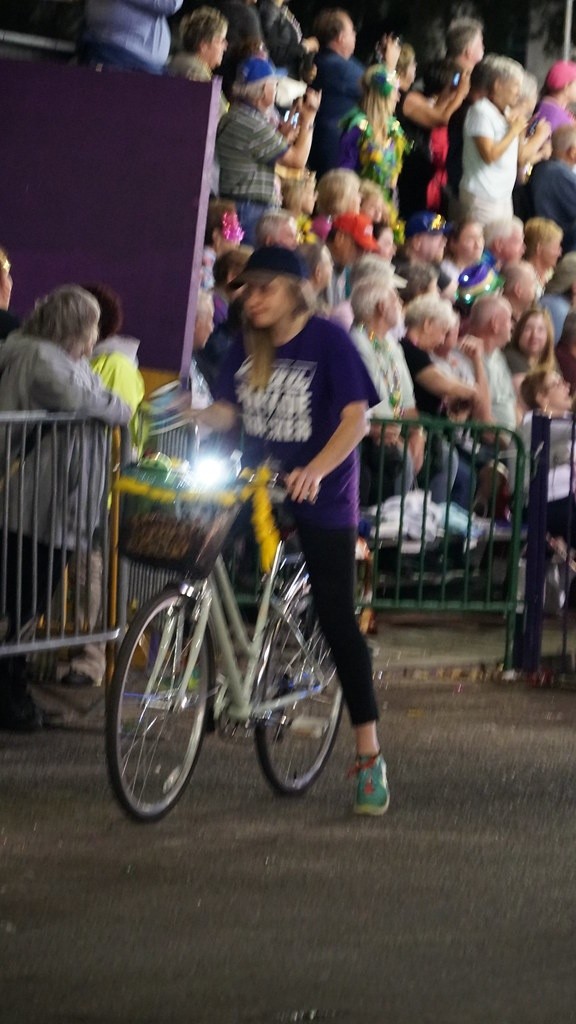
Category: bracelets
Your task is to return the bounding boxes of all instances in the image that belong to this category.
[301,124,313,129]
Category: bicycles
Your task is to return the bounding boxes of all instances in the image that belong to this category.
[100,416,378,826]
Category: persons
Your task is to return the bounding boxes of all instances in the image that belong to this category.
[0,247,147,734]
[74,0,576,620]
[175,245,389,817]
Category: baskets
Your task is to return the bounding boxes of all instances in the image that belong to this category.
[119,463,243,579]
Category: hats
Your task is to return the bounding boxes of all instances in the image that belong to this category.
[404,212,445,236]
[352,256,407,289]
[331,213,379,251]
[455,263,507,305]
[545,60,576,90]
[224,246,311,293]
[236,57,288,84]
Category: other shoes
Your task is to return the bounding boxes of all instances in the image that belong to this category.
[0,672,47,732]
[61,672,91,687]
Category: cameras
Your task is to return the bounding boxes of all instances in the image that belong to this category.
[291,94,306,112]
[453,71,462,87]
[525,119,539,138]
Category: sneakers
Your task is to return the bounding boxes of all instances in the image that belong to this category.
[184,666,203,692]
[346,747,391,815]
